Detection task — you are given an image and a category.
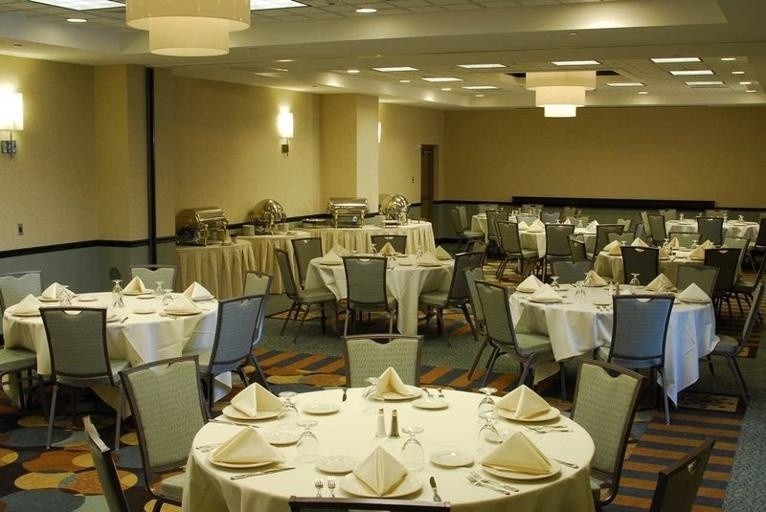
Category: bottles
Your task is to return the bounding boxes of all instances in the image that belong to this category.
[375,408,386,437]
[388,410,400,439]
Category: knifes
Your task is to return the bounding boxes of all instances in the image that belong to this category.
[429,476,442,502]
[209,418,259,429]
[230,468,294,481]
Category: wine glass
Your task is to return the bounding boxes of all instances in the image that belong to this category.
[339,244,424,267]
[58,279,174,312]
[493,207,745,299]
[294,420,320,462]
[477,410,500,449]
[274,377,503,471]
[400,425,425,465]
[476,387,498,427]
[277,391,299,426]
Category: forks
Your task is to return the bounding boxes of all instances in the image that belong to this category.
[465,475,511,496]
[328,472,337,498]
[315,479,325,497]
[472,471,519,492]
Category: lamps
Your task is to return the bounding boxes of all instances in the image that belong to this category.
[277,113,296,157]
[0,93,24,153]
[126,1,250,57]
[526,71,598,118]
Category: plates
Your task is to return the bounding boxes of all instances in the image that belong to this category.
[338,472,422,498]
[377,385,422,400]
[303,402,339,414]
[374,215,386,228]
[318,252,452,269]
[279,223,290,233]
[482,457,560,481]
[268,425,297,446]
[242,225,255,236]
[477,212,757,306]
[412,398,448,409]
[211,228,229,240]
[221,403,285,420]
[11,289,215,323]
[497,406,560,422]
[209,444,278,469]
[429,450,475,467]
